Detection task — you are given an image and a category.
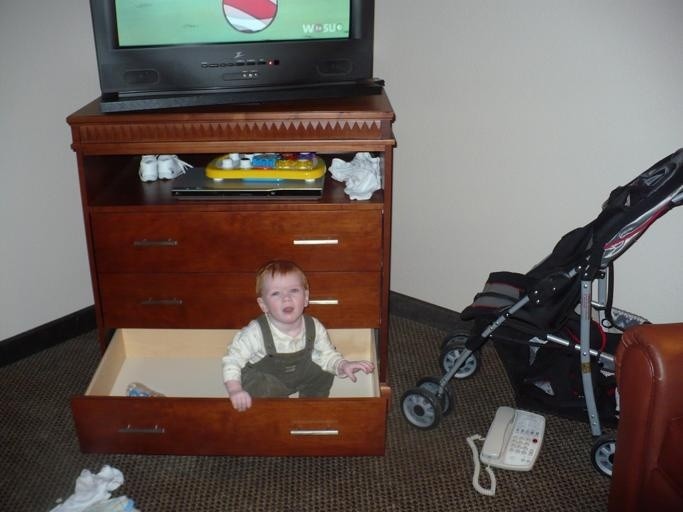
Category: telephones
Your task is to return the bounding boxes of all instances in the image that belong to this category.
[480,405,547,472]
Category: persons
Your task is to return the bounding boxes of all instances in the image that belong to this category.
[220,258,375,414]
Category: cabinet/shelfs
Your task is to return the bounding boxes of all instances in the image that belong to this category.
[66,88,398,457]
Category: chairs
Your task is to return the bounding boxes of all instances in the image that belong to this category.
[608,323,683,512]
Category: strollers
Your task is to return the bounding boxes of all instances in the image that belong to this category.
[399,143,683,478]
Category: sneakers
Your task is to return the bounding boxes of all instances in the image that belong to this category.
[138,155,193,182]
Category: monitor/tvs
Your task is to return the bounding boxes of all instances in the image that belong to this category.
[89,0,386,114]
